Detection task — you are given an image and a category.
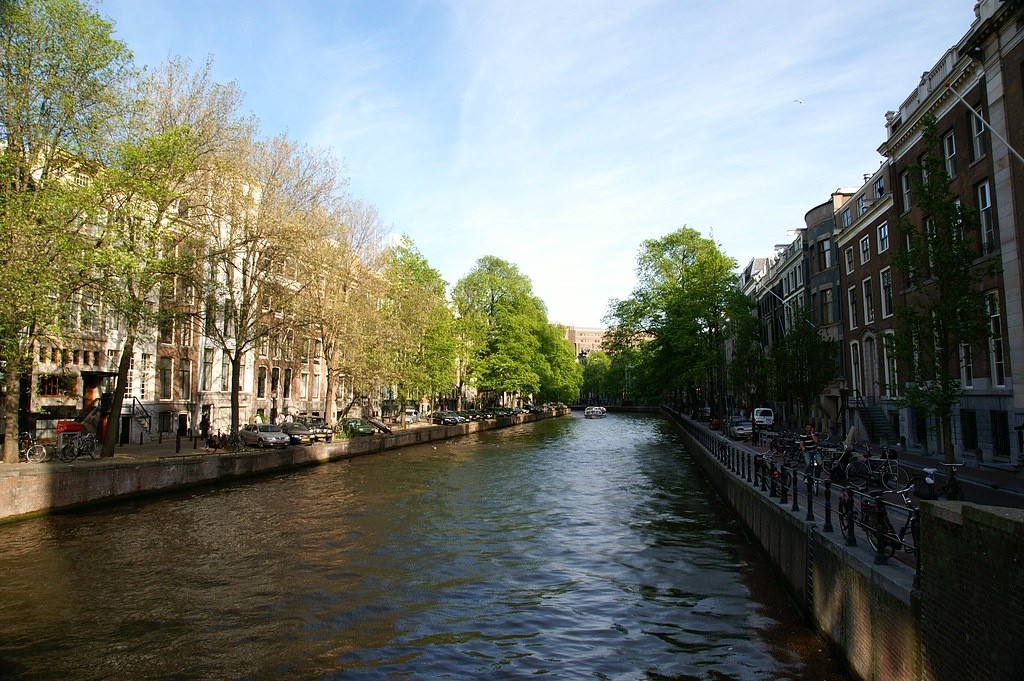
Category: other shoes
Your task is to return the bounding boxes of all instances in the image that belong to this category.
[815,480,821,483]
[804,478,808,484]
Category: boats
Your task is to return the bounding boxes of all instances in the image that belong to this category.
[585,406,608,419]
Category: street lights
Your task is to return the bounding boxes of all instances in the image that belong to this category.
[271,393,276,424]
[749,384,761,446]
[837,376,849,442]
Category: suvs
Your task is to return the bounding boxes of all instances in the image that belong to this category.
[296,416,333,443]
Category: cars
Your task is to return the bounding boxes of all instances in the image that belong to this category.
[338,418,375,436]
[239,424,291,447]
[278,422,317,443]
[396,408,420,424]
[444,402,567,423]
[723,415,753,440]
[427,412,457,426]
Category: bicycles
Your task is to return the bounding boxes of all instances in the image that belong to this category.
[838,482,875,546]
[204,429,247,453]
[902,460,969,505]
[18,431,107,463]
[867,484,920,562]
[756,430,910,495]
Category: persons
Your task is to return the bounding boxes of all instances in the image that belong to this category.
[249,412,263,424]
[735,404,752,416]
[274,411,300,425]
[800,423,823,484]
[199,414,210,440]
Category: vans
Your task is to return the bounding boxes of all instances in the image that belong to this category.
[750,408,775,430]
